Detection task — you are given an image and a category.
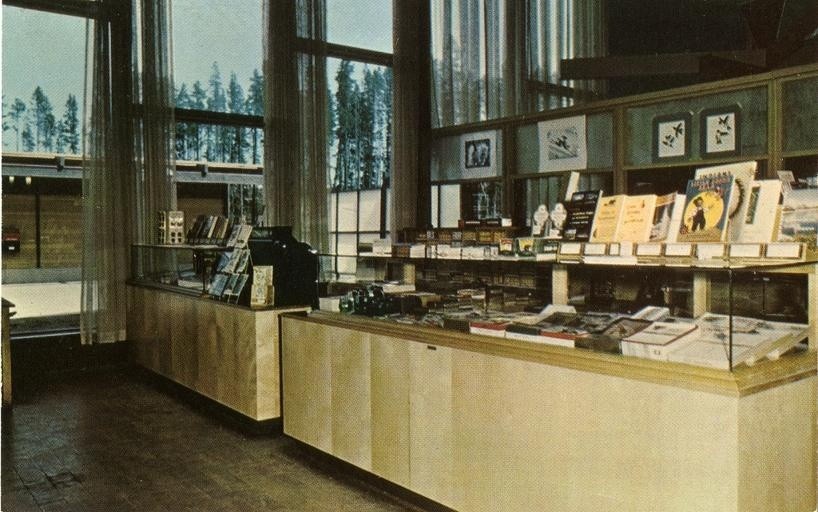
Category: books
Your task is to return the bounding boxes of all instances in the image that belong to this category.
[564,161,818,246]
[320,280,810,370]
[158,209,276,309]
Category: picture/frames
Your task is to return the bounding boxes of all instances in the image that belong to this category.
[651,103,741,167]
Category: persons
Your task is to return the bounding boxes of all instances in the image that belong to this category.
[465,142,478,167]
[475,142,490,167]
